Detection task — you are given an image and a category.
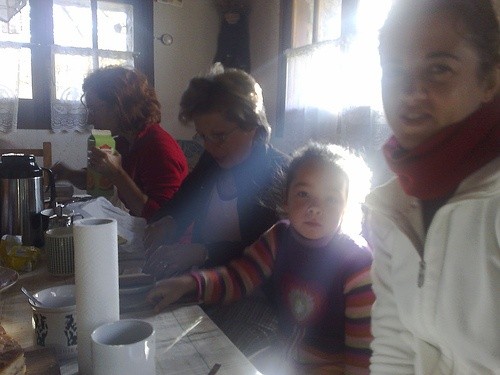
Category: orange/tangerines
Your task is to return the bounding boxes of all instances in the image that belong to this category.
[100,175,112,190]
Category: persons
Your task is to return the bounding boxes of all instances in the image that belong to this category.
[52,69,194,247]
[145,62,281,277]
[143,141,375,375]
[363,0,500,375]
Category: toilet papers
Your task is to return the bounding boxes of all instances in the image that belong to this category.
[70,217,122,375]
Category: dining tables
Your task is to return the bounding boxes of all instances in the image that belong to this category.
[0,173,262,375]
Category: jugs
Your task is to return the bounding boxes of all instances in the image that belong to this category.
[0,152,57,249]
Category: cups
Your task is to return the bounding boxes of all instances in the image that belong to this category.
[44,227,74,277]
[40,208,54,239]
[28,284,78,362]
[90,319,157,375]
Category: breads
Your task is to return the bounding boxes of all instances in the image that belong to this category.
[0,349,27,375]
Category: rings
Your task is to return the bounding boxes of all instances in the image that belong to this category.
[163,263,168,268]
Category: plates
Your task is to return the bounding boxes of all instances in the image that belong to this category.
[0,266,20,294]
[118,260,157,294]
